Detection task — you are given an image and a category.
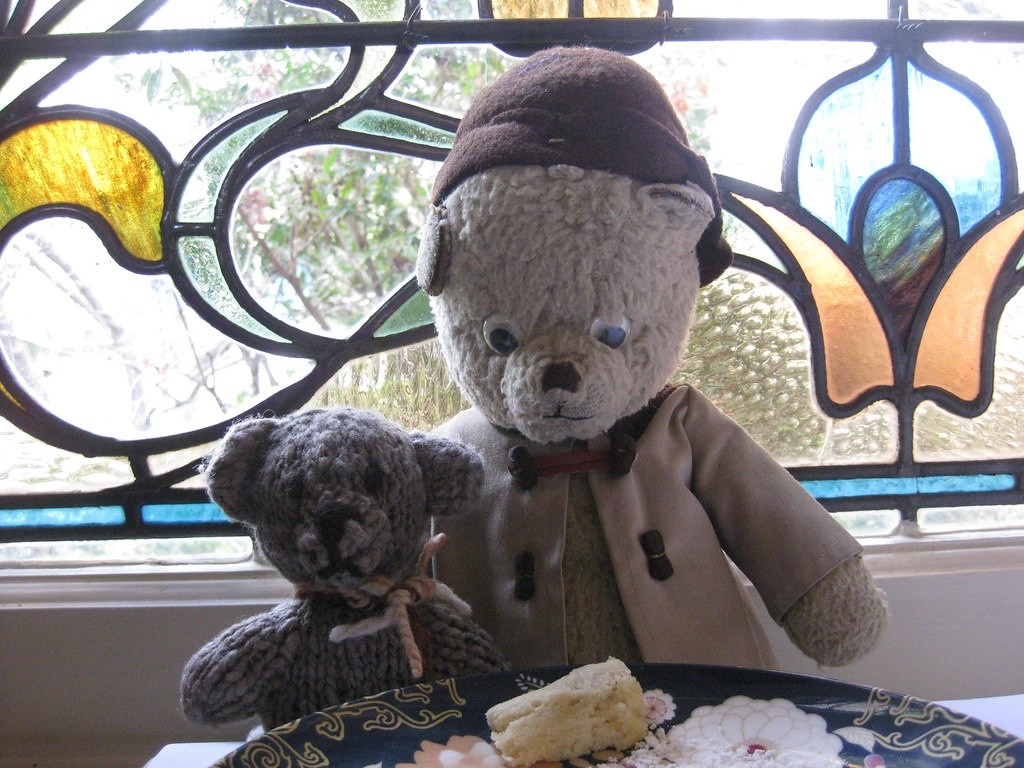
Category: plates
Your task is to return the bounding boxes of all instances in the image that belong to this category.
[190,662,1024,767]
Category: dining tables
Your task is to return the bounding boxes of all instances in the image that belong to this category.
[140,692,1024,767]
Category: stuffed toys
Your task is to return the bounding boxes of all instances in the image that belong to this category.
[180,408,508,733]
[387,47,888,671]
